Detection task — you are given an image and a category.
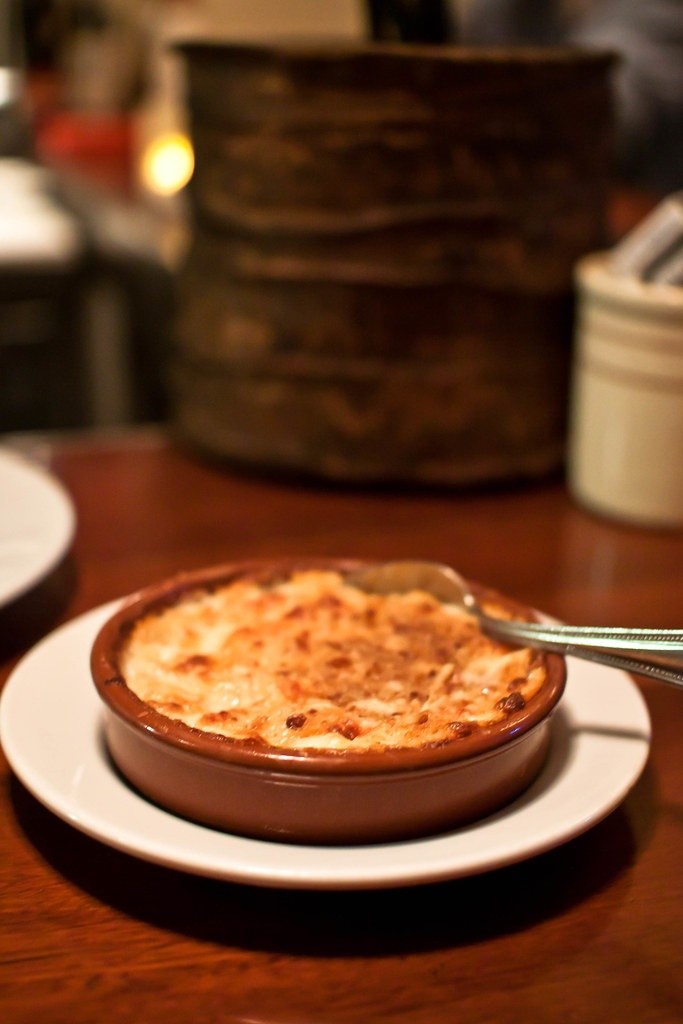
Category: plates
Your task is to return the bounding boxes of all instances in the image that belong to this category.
[1,446,77,611]
[0,589,653,892]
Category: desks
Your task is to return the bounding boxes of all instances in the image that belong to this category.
[0,426,683,1024]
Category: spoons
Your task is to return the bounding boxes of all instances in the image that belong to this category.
[348,552,683,702]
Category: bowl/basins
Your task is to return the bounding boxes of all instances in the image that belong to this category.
[92,554,563,838]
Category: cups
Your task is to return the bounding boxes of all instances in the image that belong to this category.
[564,250,683,527]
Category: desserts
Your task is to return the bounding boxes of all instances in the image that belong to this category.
[118,568,546,749]
